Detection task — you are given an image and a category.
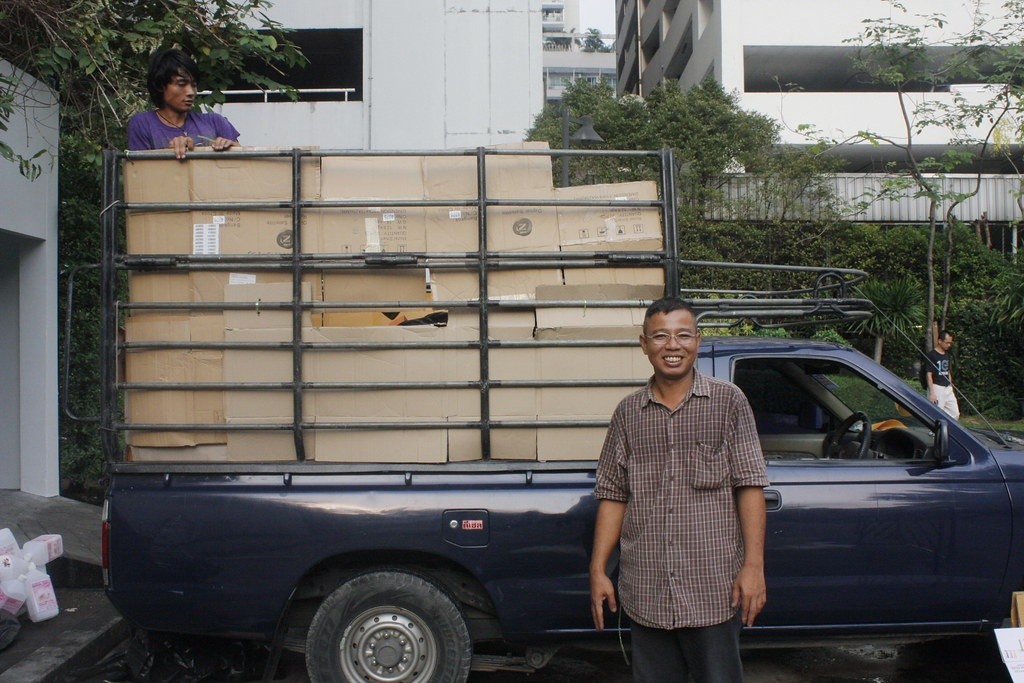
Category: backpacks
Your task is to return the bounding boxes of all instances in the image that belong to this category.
[919,362,929,390]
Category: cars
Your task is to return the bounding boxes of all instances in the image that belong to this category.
[738,359,864,464]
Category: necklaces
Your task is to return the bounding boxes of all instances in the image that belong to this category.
[155,110,188,137]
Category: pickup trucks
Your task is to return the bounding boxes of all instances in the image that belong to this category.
[99,337,1024,683]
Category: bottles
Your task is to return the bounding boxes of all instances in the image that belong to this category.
[0,527,64,621]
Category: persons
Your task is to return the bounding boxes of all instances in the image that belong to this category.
[927,330,960,421]
[590,298,770,683]
[129,46,240,160]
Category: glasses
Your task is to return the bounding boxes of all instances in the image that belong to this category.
[645,332,697,345]
